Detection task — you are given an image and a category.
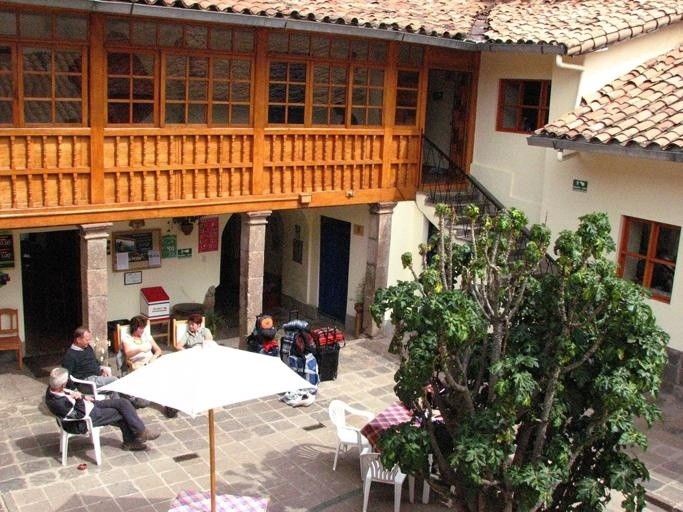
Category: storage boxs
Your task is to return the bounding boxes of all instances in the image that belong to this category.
[141,286,170,318]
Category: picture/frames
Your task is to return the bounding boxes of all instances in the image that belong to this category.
[124,271,142,285]
[112,227,161,272]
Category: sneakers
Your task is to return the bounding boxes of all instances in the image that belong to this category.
[134,429,161,442]
[123,438,147,451]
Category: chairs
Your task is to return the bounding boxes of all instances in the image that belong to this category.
[0,308,23,370]
[328,399,374,470]
[172,317,207,351]
[70,364,112,400]
[117,320,151,379]
[51,388,102,467]
[361,453,416,512]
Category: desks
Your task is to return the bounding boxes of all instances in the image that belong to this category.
[141,313,170,349]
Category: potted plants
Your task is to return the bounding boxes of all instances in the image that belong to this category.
[207,312,226,339]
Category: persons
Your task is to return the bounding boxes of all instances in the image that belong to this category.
[174,315,214,350]
[121,315,179,418]
[46,368,162,452]
[62,327,146,409]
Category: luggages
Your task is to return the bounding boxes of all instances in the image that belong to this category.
[280,310,300,363]
[288,353,316,386]
[255,313,279,356]
[313,344,340,381]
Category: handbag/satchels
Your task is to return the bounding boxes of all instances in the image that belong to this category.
[313,327,346,350]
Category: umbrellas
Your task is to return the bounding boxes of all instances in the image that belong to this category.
[95,340,318,512]
